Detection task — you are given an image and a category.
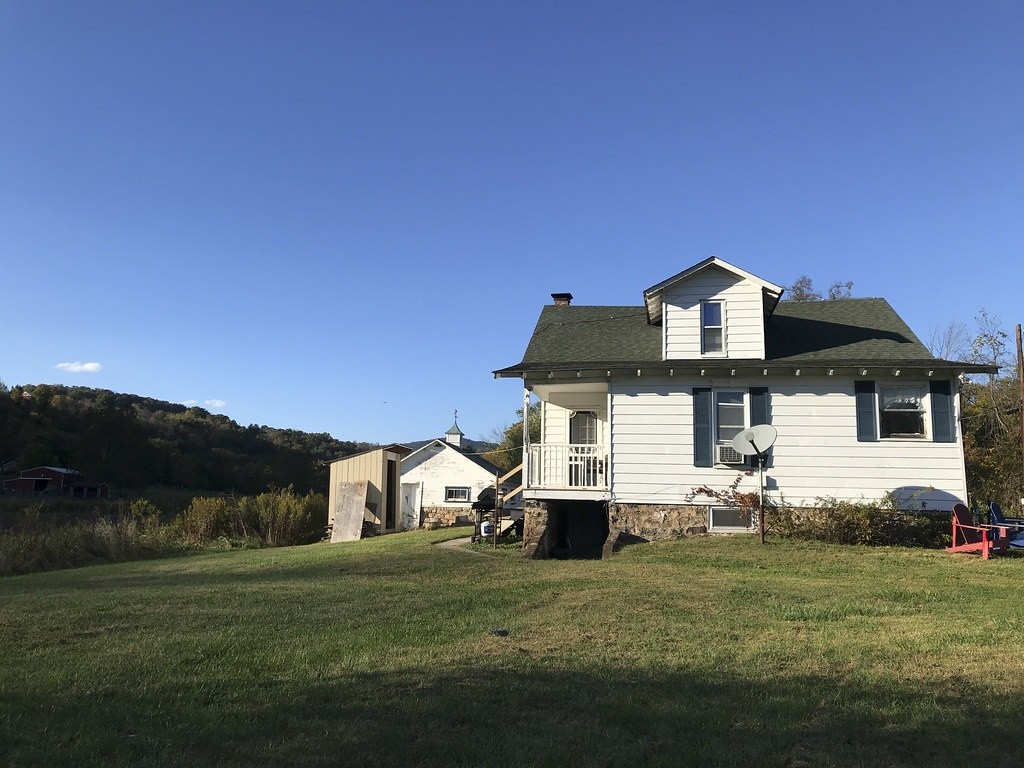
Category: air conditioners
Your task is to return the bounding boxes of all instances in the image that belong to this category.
[715,444,744,464]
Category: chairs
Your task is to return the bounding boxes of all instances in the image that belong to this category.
[944,503,1014,560]
[989,502,1024,550]
